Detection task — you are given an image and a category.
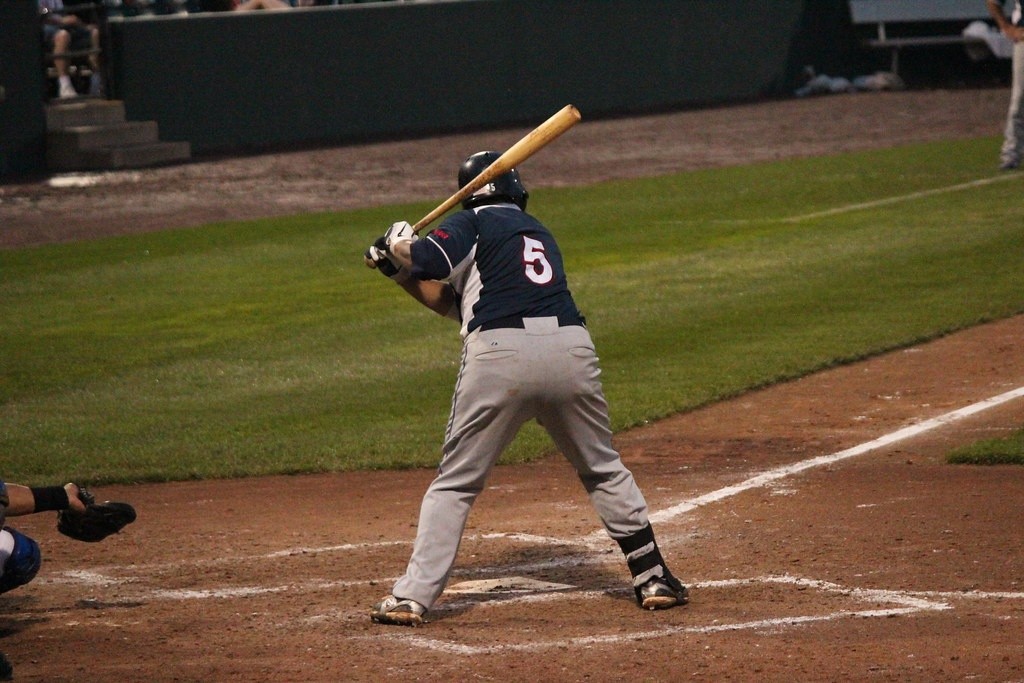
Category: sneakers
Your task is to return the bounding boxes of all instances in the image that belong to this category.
[370,595,426,624]
[640,576,690,607]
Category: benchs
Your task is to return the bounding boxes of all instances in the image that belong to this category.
[850,0,1014,74]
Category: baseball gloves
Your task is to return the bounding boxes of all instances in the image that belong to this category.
[52,487,141,543]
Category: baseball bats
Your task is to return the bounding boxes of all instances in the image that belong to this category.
[363,104,583,269]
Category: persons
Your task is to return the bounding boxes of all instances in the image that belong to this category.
[37,0,103,98]
[369,153,689,621]
[0,482,136,683]
[987,0,1024,171]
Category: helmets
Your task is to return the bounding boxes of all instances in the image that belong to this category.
[457,150,529,207]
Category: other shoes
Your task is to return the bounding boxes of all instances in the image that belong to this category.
[1001,163,1019,171]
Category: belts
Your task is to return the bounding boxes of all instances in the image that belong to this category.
[479,315,587,333]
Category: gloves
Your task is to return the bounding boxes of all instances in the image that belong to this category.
[364,221,420,279]
